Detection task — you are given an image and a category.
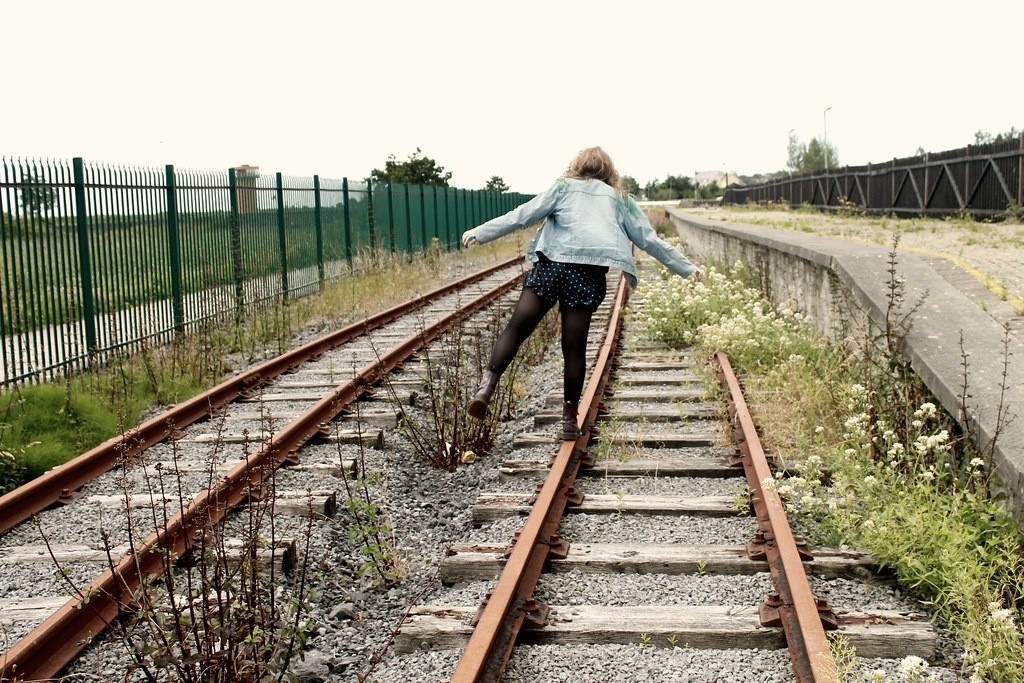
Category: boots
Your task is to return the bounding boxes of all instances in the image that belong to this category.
[559,400,583,441]
[468,370,498,421]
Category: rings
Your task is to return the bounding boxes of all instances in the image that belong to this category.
[467,235,475,241]
[696,271,701,277]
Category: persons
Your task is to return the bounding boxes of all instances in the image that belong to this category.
[458,146,705,443]
